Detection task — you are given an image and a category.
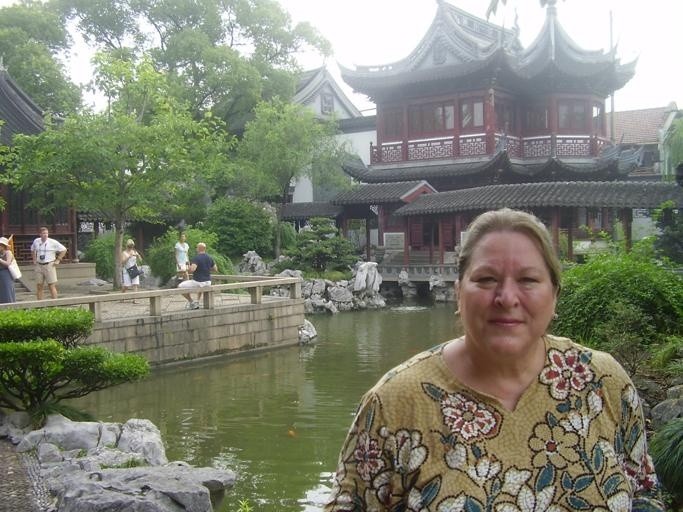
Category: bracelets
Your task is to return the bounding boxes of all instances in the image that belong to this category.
[56,256,62,261]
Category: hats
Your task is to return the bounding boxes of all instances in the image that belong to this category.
[0,237,8,246]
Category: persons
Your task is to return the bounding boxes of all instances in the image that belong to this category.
[29,226,67,300]
[119,239,144,304]
[0,237,16,303]
[319,206,665,511]
[172,232,189,288]
[175,241,217,311]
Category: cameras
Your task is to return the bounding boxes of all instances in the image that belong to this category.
[40,255,45,259]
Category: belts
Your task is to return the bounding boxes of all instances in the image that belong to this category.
[39,262,48,265]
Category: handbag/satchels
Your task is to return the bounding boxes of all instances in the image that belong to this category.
[176,264,186,272]
[7,259,21,280]
[126,266,140,279]
